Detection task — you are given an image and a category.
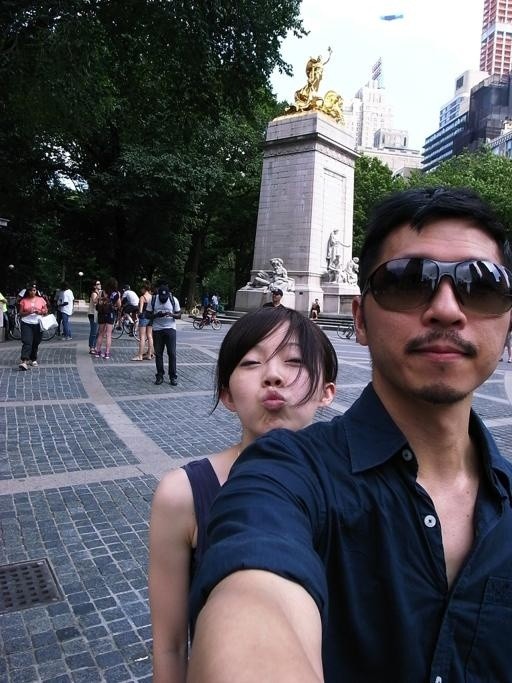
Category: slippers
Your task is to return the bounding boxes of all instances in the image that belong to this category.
[89,350,96,354]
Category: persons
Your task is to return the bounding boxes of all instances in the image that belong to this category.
[0,280,222,386]
[185,183,512,683]
[146,308,343,683]
[294,44,334,102]
[310,298,320,319]
[262,289,284,307]
[499,330,512,363]
[325,228,360,284]
[246,257,287,286]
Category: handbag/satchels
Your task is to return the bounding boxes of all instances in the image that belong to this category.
[38,311,59,333]
[98,297,111,314]
[139,295,147,310]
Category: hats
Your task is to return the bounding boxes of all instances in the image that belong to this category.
[271,289,283,296]
[158,287,168,303]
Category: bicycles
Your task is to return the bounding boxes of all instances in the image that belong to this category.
[110,306,149,343]
[191,302,200,316]
[337,320,355,340]
[192,308,223,330]
[6,302,58,341]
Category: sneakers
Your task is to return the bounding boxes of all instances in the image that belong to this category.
[171,379,177,385]
[61,338,70,341]
[131,351,157,361]
[30,361,38,367]
[94,351,105,358]
[155,377,163,384]
[104,352,112,358]
[18,363,28,370]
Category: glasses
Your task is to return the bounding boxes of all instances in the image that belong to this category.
[358,256,511,317]
